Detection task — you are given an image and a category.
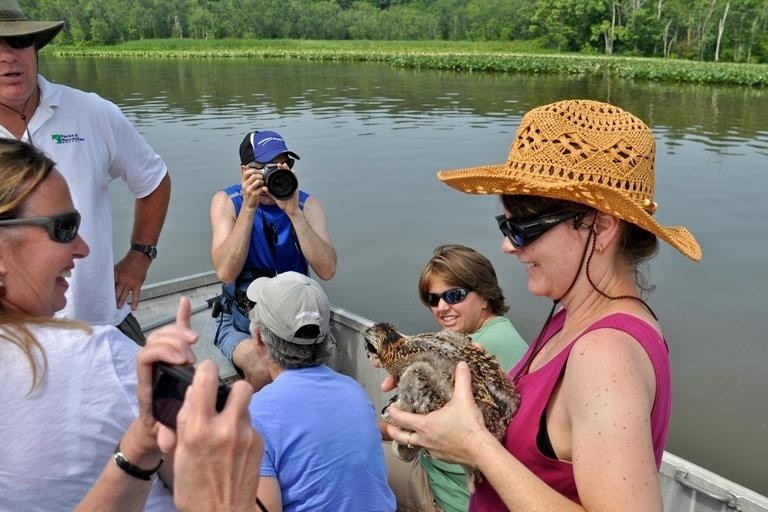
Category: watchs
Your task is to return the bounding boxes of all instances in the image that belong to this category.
[127,241,159,262]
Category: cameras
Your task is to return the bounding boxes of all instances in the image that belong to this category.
[257,163,298,201]
[152,360,230,431]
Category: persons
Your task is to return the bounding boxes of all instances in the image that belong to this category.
[377,242,533,511]
[0,0,173,347]
[245,268,401,512]
[368,97,705,512]
[69,291,267,510]
[0,137,181,512]
[207,129,338,396]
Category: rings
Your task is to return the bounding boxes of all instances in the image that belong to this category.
[406,429,415,450]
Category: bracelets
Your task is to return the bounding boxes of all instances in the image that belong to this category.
[113,441,165,484]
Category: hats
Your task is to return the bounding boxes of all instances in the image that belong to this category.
[0,0,65,52]
[239,132,300,163]
[437,99,702,262]
[247,270,330,345]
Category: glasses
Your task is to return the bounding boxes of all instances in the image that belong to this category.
[495,211,584,249]
[4,33,35,48]
[0,210,81,244]
[423,286,472,308]
[283,160,294,169]
